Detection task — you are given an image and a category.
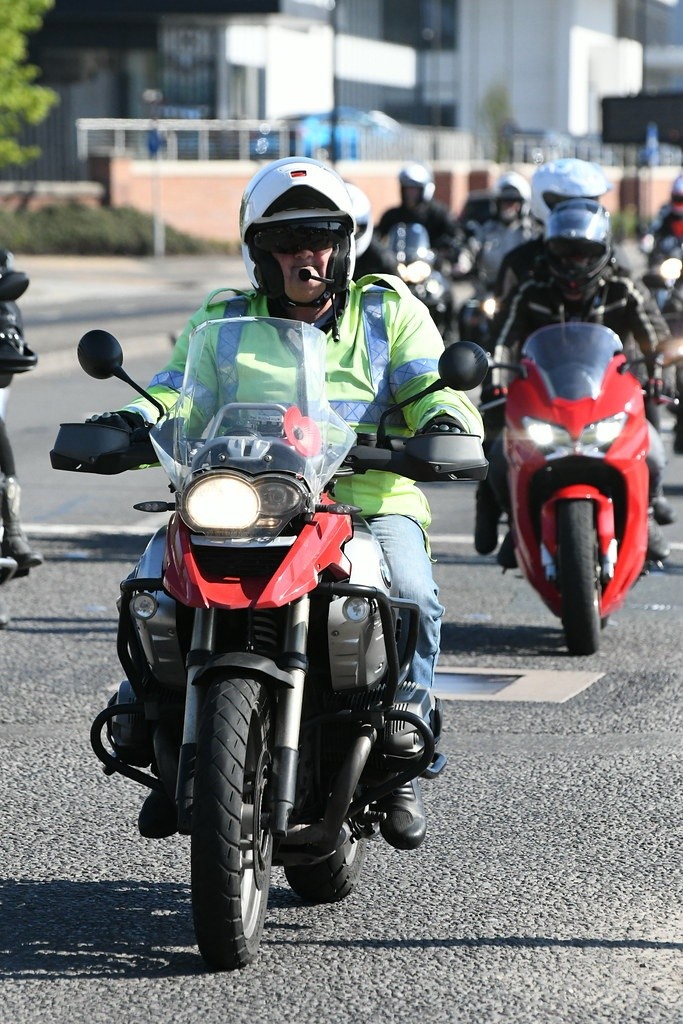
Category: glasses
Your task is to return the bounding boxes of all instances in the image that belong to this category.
[254,222,349,254]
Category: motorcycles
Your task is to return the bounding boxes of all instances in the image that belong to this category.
[635,220,683,326]
[475,321,683,658]
[375,222,455,349]
[450,220,530,352]
[49,328,492,974]
[1,271,38,586]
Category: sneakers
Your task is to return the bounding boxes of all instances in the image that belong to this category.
[379,776,426,850]
[138,790,179,839]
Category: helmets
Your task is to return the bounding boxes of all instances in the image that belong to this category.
[398,159,436,204]
[345,183,374,258]
[486,170,532,219]
[542,197,616,305]
[238,155,356,297]
[530,157,613,227]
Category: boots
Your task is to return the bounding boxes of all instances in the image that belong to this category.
[3,474,32,561]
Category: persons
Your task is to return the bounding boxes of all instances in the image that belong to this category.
[480,196,672,566]
[85,156,490,851]
[328,151,679,459]
[470,158,677,554]
[0,243,46,578]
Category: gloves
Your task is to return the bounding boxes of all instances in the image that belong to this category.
[480,384,509,404]
[642,377,680,406]
[85,412,134,434]
[416,424,467,434]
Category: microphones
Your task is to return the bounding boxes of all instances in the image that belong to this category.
[298,269,335,285]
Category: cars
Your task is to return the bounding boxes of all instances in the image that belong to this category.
[505,128,596,166]
[251,107,415,161]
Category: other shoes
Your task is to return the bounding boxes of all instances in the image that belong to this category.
[474,480,502,555]
[652,495,677,524]
[647,516,669,561]
